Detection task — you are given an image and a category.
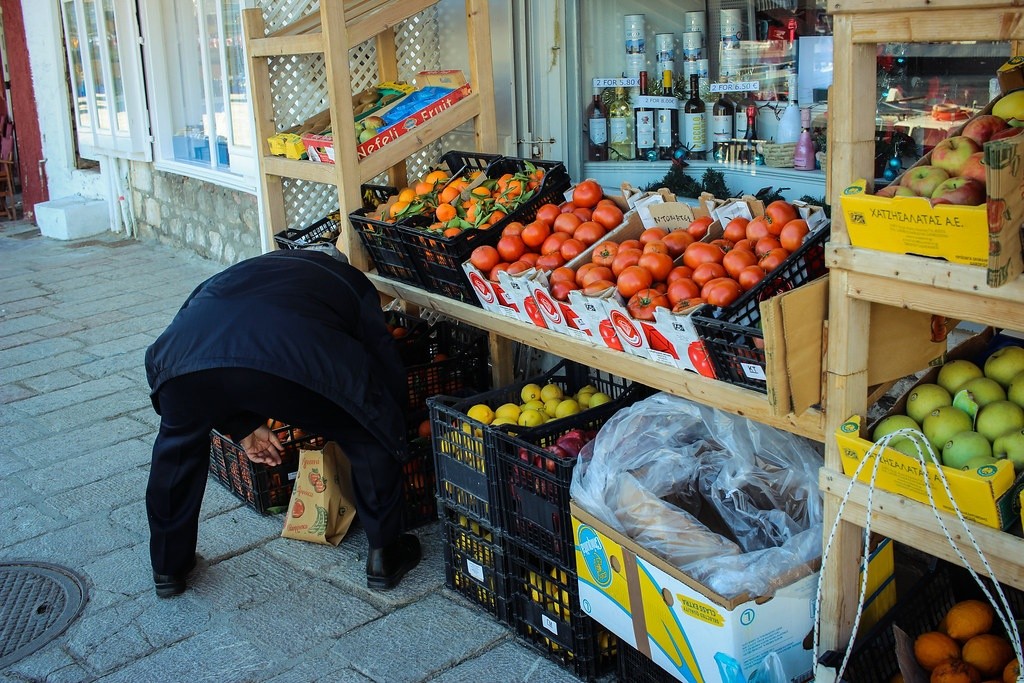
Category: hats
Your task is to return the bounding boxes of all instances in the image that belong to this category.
[304,242,349,264]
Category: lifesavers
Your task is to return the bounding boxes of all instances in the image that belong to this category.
[931,102,974,120]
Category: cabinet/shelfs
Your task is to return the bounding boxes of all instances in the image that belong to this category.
[240,0,1024,683]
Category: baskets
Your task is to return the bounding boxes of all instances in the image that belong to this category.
[763,141,796,168]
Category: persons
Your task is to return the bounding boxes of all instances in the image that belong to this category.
[144,245,422,597]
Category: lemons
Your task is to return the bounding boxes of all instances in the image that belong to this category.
[447,485,618,658]
[440,384,613,473]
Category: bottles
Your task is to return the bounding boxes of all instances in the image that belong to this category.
[685,74,706,160]
[586,78,608,162]
[634,71,655,160]
[713,76,734,162]
[610,78,630,161]
[736,80,759,164]
[775,74,815,170]
[658,70,678,160]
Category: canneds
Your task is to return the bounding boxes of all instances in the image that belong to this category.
[623,7,744,101]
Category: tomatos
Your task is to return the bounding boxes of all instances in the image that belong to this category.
[470,179,811,372]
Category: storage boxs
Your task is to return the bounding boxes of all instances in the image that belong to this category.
[208,82,1024,683]
[34,194,110,240]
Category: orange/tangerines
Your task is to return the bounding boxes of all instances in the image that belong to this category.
[364,170,546,265]
[892,600,1020,683]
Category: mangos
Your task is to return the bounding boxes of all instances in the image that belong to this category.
[354,116,387,144]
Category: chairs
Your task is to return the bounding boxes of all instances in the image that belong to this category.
[0,158,17,219]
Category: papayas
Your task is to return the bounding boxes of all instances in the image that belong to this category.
[992,90,1024,121]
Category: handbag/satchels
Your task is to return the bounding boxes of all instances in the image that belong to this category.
[281,439,355,546]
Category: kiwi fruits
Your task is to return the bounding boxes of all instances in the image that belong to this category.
[295,93,398,135]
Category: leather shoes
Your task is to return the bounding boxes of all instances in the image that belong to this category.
[150,555,199,596]
[367,533,422,590]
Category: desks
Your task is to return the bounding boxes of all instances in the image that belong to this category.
[173,135,228,165]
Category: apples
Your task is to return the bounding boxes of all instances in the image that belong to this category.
[385,325,462,515]
[873,346,1024,470]
[510,430,595,553]
[210,419,324,503]
[874,115,1024,206]
